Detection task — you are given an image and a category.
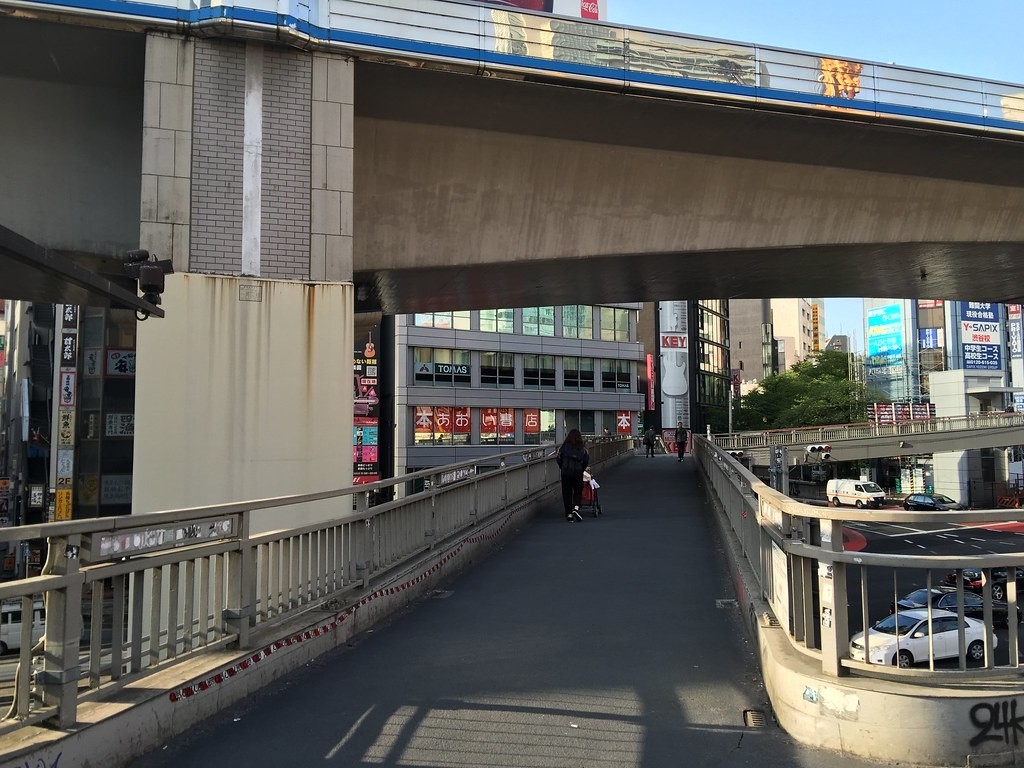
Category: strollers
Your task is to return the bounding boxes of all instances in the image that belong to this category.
[579,466,602,518]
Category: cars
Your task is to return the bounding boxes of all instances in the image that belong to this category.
[888,585,1023,631]
[944,565,1024,601]
[902,492,972,511]
[848,607,1000,668]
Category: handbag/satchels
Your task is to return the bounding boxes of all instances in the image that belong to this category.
[643,437,650,445]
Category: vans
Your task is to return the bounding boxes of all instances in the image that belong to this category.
[0,600,85,657]
[826,478,887,509]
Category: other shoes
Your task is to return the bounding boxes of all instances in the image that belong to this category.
[568,517,575,522]
[572,511,584,521]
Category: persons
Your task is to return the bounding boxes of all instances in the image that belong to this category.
[643,425,656,458]
[1005,403,1014,413]
[556,429,589,522]
[675,422,688,461]
[603,428,611,435]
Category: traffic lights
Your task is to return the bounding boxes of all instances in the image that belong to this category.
[806,444,831,452]
[821,453,831,460]
[725,451,743,458]
